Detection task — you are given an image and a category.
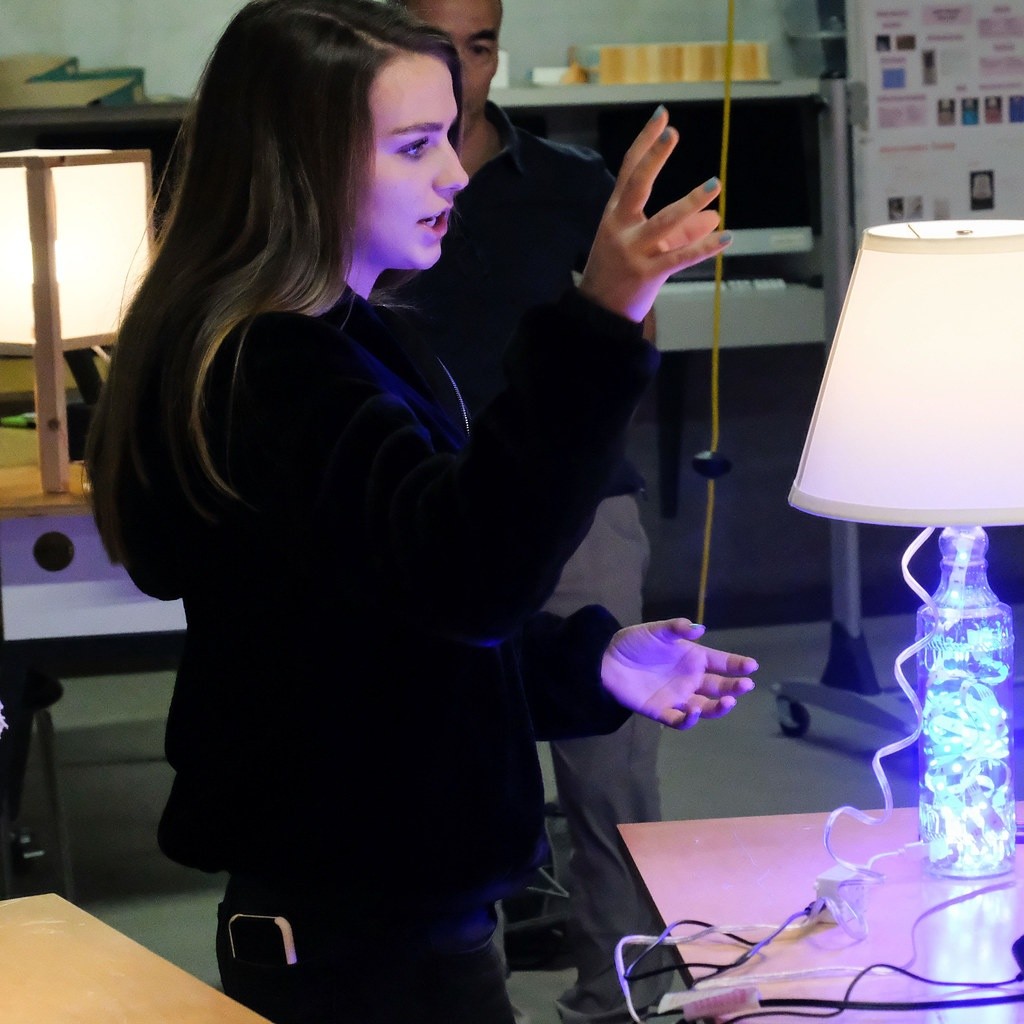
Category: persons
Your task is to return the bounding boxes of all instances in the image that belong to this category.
[91,0,759,1024]
[371,0,679,1024]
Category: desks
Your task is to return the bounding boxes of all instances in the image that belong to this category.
[0,893,274,1024]
[616,800,1024,1024]
[0,518,188,903]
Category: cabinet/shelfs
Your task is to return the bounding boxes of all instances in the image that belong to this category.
[485,77,827,776]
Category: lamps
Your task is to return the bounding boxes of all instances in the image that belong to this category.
[786,217,1024,882]
[1,148,157,514]
